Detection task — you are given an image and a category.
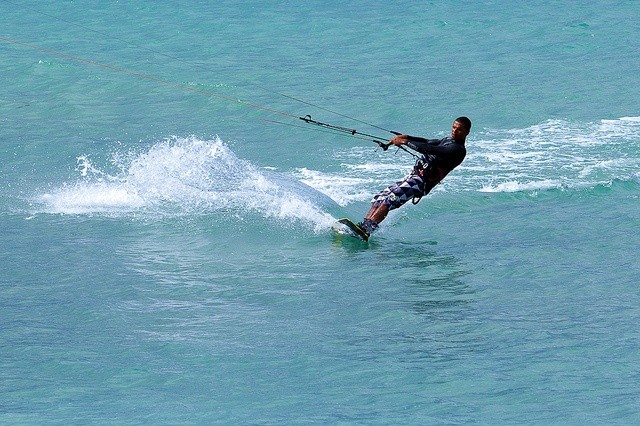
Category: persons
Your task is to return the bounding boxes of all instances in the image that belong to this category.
[357,116,471,232]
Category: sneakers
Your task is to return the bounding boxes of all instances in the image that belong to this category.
[357,218,379,236]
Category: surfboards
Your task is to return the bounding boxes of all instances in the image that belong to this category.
[331,217,369,241]
